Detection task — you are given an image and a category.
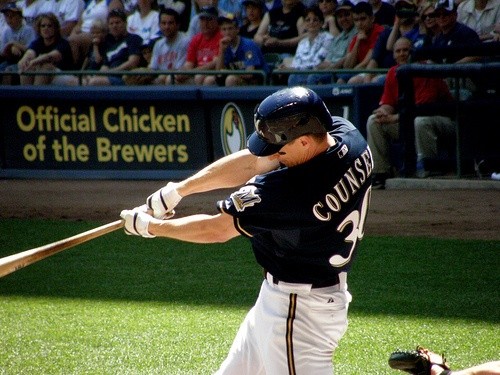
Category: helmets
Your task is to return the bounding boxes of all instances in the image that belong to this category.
[245,85,333,157]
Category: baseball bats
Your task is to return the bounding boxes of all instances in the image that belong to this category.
[0,210,175,277]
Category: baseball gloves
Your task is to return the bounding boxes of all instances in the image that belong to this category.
[389,347,450,374]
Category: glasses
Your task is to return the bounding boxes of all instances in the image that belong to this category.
[421,11,434,20]
[113,7,125,17]
[40,23,53,28]
[305,16,318,22]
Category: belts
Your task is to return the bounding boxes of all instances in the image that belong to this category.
[264,272,340,295]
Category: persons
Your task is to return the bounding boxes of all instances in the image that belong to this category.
[0,0,16,25]
[108,0,124,12]
[137,8,190,86]
[238,0,262,38]
[127,0,159,44]
[278,6,334,85]
[204,11,265,86]
[368,0,395,26]
[457,0,500,43]
[189,0,223,34]
[318,0,340,35]
[389,345,500,375]
[420,2,441,34]
[91,9,142,86]
[73,0,108,34]
[428,0,484,63]
[17,0,45,25]
[37,0,84,35]
[81,21,104,86]
[18,12,74,84]
[343,1,385,83]
[254,0,305,53]
[120,83,375,375]
[365,0,422,83]
[308,0,358,84]
[1,7,35,63]
[175,5,224,86]
[366,38,457,186]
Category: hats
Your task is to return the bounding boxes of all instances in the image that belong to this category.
[0,2,22,13]
[241,0,265,8]
[394,0,418,7]
[434,0,455,14]
[198,5,219,18]
[334,0,354,13]
[218,12,239,27]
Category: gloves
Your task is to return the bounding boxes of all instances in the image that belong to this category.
[119,209,157,239]
[146,181,183,219]
